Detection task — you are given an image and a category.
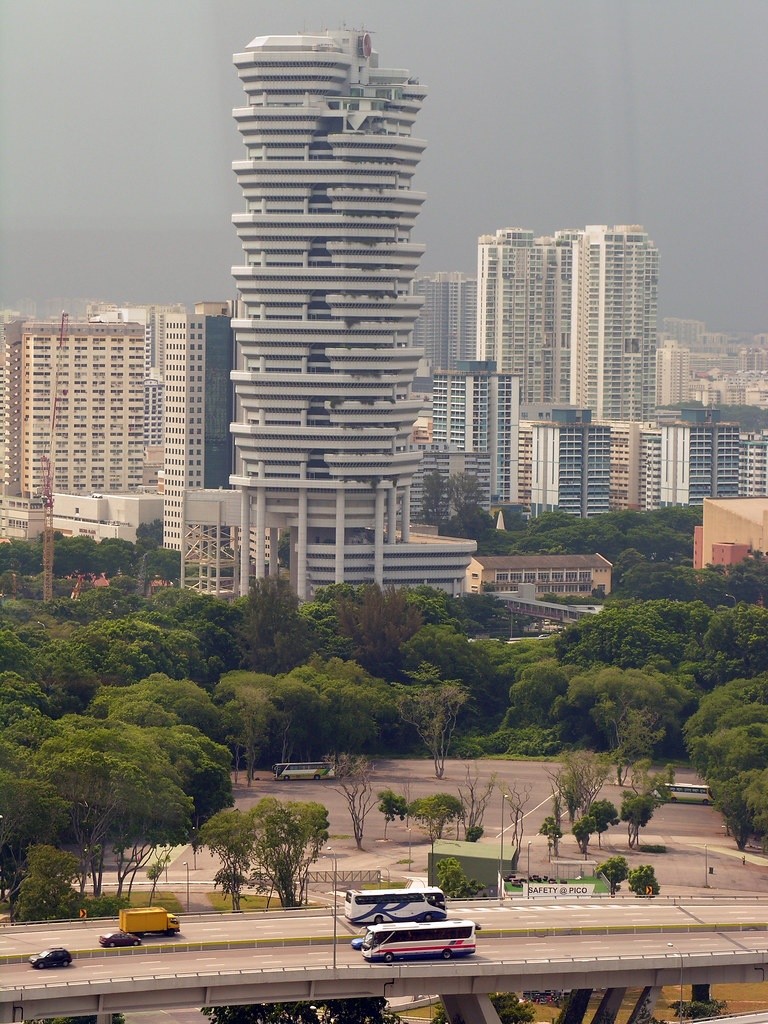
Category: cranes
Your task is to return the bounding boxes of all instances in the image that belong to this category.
[40,309,68,601]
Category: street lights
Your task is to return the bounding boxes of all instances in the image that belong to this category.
[377,866,390,889]
[327,846,337,968]
[528,841,532,899]
[409,829,411,871]
[704,843,707,886]
[667,943,683,1024]
[500,794,508,900]
[505,605,512,637]
[183,862,189,912]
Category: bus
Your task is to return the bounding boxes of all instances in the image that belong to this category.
[345,888,447,924]
[358,920,477,963]
[272,762,336,781]
[653,782,716,805]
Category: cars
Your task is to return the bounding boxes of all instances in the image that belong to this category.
[351,937,365,950]
[445,918,481,930]
[99,931,142,947]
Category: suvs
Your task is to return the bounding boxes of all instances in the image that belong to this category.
[27,947,72,969]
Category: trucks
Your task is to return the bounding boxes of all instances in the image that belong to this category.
[119,906,180,938]
